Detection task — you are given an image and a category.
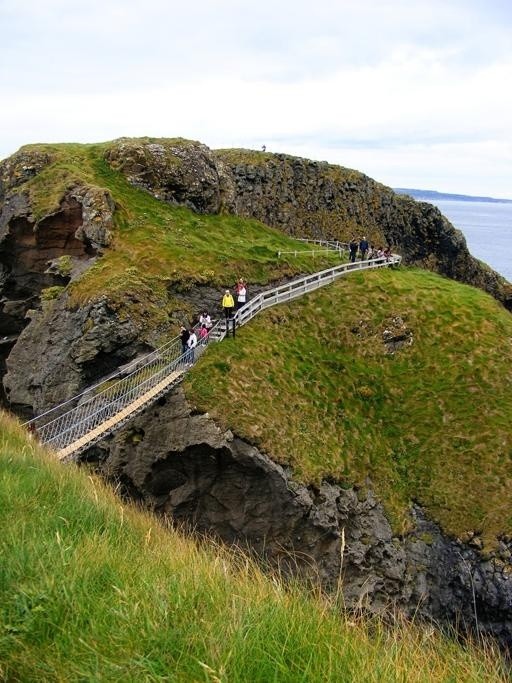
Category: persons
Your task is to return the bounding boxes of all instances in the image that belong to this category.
[349,236,392,264]
[180,277,249,369]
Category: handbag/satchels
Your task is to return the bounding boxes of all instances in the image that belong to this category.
[191,340,197,348]
[205,321,213,329]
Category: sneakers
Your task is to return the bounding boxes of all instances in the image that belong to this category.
[184,362,194,368]
